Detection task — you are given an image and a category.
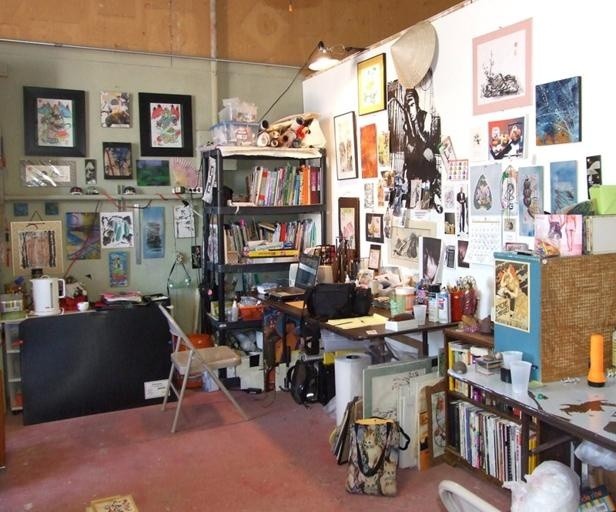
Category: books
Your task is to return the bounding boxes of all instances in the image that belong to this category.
[445,337,616,511]
[207,163,322,264]
[94,291,144,311]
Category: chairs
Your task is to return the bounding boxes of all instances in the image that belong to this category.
[152,298,252,435]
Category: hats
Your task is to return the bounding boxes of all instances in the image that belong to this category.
[391,22,436,89]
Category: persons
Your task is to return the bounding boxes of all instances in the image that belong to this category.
[500,272,519,318]
[402,88,431,151]
[423,238,440,284]
[457,187,467,234]
[547,214,576,253]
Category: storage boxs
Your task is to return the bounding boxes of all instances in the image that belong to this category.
[208,119,261,149]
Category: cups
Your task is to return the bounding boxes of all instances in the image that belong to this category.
[76,300,90,311]
[502,350,522,369]
[508,360,532,395]
[413,305,427,325]
[389,295,406,318]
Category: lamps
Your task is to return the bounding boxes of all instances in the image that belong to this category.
[305,42,367,73]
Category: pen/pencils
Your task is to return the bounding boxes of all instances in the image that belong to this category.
[337,322,352,326]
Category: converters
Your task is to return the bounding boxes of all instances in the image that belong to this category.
[172,186,185,193]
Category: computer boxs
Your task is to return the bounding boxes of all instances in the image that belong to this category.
[318,359,335,406]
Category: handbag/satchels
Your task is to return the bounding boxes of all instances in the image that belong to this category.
[286,360,317,404]
[307,282,371,317]
[346,418,399,497]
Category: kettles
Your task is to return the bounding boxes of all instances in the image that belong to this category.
[30,275,64,314]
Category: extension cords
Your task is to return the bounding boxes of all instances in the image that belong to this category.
[172,187,203,194]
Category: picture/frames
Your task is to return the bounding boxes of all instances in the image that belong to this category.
[387,223,438,268]
[536,76,582,146]
[518,166,544,236]
[365,212,383,244]
[334,110,358,181]
[362,182,375,209]
[550,161,578,215]
[360,124,379,178]
[534,214,582,256]
[5,84,197,277]
[385,67,442,223]
[379,130,390,167]
[368,245,382,270]
[338,196,361,263]
[356,53,387,117]
[471,18,533,117]
[417,235,443,282]
[375,170,393,209]
[486,116,529,161]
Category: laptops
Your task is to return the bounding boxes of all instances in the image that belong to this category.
[266,253,320,301]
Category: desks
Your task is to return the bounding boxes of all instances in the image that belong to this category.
[538,253,614,512]
[250,282,456,366]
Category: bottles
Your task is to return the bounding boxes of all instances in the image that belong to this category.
[232,300,238,321]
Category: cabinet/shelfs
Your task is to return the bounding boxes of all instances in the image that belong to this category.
[2,294,172,421]
[442,322,541,494]
[198,143,329,390]
